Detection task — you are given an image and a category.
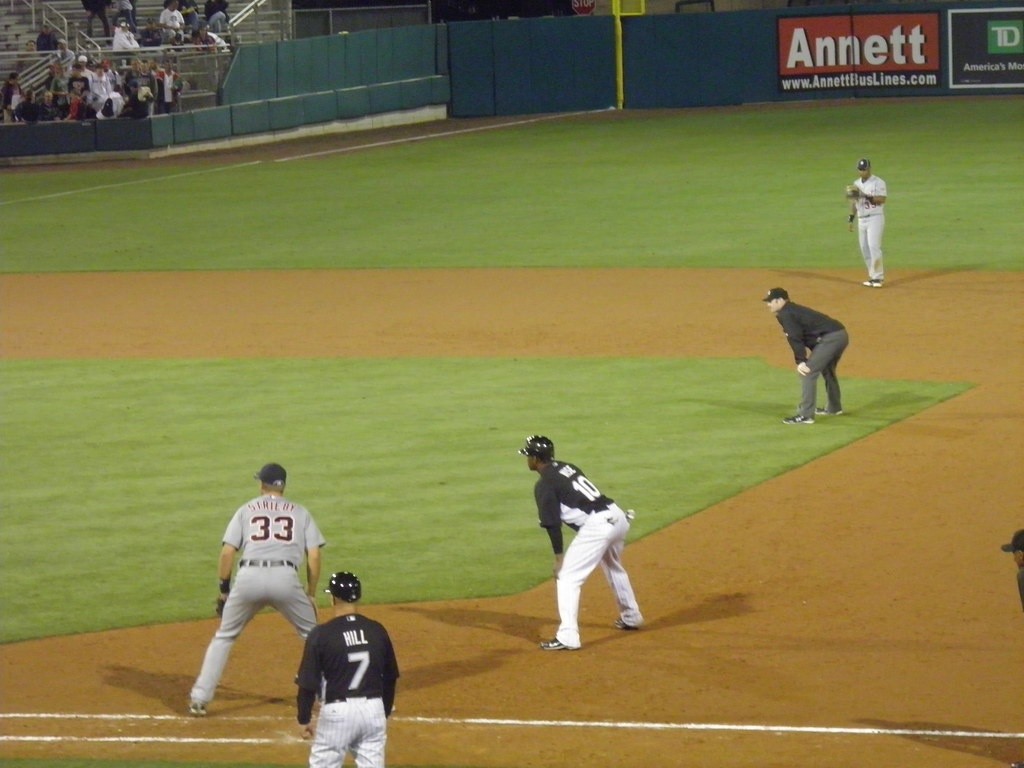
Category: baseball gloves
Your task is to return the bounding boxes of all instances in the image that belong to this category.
[215,598,225,618]
[846,185,860,198]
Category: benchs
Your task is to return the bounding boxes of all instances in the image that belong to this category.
[0,0,289,124]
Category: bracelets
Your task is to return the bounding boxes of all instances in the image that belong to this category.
[218,576,231,594]
[848,215,854,223]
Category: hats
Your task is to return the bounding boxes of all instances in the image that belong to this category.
[126,80,138,89]
[762,288,790,302]
[78,55,87,62]
[254,464,287,485]
[858,159,871,170]
[1000,530,1024,554]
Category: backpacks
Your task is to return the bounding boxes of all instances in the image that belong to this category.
[101,98,114,118]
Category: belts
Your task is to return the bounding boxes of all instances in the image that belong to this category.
[331,695,380,704]
[239,558,299,573]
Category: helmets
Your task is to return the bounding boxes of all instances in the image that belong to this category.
[323,572,362,603]
[517,435,555,458]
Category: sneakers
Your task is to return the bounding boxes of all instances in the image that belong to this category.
[782,415,815,425]
[863,280,883,287]
[615,619,639,630]
[541,638,581,650]
[815,406,843,415]
[190,704,206,715]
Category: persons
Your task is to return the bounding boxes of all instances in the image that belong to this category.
[0,0,230,122]
[294,572,401,768]
[187,464,328,717]
[846,159,887,287]
[517,433,645,650]
[762,287,849,424]
[1001,529,1024,612]
